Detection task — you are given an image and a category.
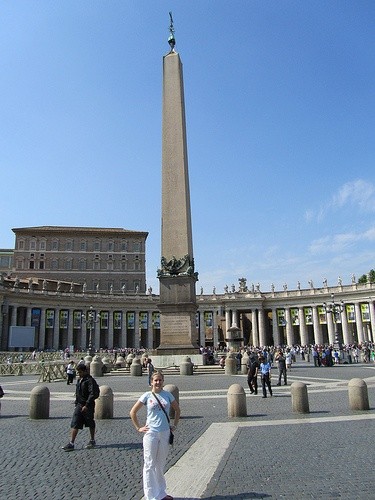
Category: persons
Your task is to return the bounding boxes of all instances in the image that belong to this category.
[61,364,100,450]
[236,345,305,368]
[130,371,180,500]
[32,350,36,361]
[143,358,154,387]
[259,357,273,398]
[219,356,225,367]
[246,357,259,395]
[20,354,24,363]
[7,356,12,364]
[200,273,371,295]
[200,346,213,364]
[274,352,287,385]
[66,361,74,385]
[13,275,152,295]
[64,346,70,360]
[79,358,85,364]
[112,347,145,353]
[311,344,375,366]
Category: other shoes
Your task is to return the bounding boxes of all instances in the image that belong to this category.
[284,384,287,385]
[262,395,267,398]
[277,383,281,386]
[250,390,255,394]
[270,393,272,396]
[62,441,74,451]
[85,440,96,449]
[161,495,173,500]
[254,392,258,394]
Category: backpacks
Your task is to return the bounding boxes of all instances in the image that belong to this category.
[92,376,100,401]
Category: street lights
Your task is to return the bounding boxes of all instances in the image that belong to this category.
[81,305,102,356]
[322,292,344,349]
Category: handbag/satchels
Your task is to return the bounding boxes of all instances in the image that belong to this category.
[169,431,174,444]
